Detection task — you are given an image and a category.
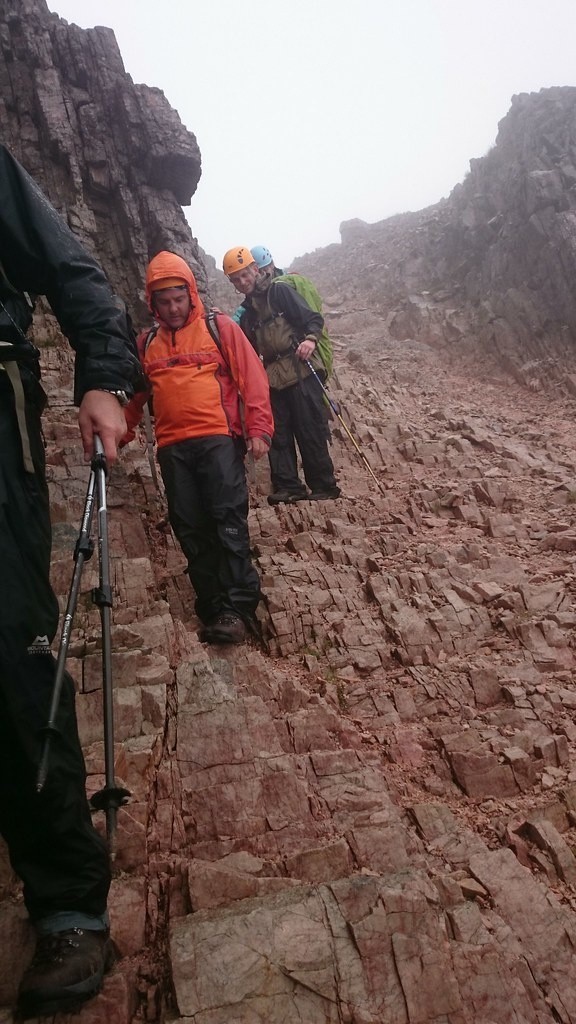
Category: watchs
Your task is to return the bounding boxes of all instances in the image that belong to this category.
[88,388,133,406]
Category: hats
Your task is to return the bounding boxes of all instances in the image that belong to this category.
[146,278,187,291]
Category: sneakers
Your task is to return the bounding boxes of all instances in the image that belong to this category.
[9,912,116,1019]
[268,485,305,502]
[301,487,340,499]
[211,607,247,645]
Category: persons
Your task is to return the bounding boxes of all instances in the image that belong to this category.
[0,140,145,1010]
[232,246,297,323]
[223,247,340,503]
[118,251,274,642]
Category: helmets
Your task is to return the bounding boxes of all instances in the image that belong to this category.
[249,244,272,270]
[219,247,254,276]
